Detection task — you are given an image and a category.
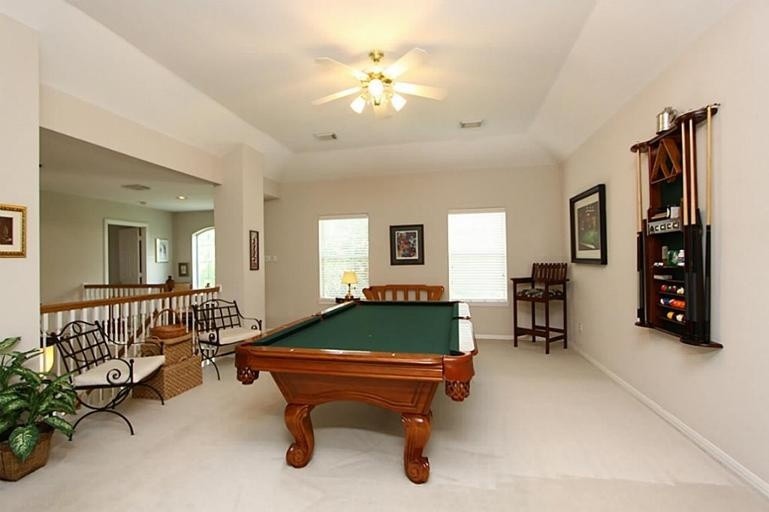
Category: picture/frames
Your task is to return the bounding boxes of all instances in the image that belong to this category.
[154,238,171,265]
[568,183,607,268]
[389,222,426,267]
[177,262,188,276]
[0,202,28,261]
[249,229,261,271]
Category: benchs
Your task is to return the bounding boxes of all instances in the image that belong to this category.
[362,283,446,303]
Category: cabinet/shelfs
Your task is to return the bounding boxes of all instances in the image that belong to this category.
[627,102,727,349]
[336,297,362,307]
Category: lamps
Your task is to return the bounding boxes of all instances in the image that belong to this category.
[340,271,357,298]
[348,76,406,115]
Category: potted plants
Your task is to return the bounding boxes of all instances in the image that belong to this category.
[0,336,76,482]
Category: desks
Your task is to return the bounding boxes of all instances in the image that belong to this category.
[231,296,479,486]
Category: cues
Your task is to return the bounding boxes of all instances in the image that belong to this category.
[636,149,641,323]
[680,106,711,344]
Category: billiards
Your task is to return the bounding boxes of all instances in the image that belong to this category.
[661,284,685,295]
[660,298,686,308]
[667,312,686,323]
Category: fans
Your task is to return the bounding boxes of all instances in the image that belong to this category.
[311,45,444,105]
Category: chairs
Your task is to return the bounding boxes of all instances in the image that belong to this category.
[45,317,167,441]
[191,297,263,383]
[511,262,571,355]
